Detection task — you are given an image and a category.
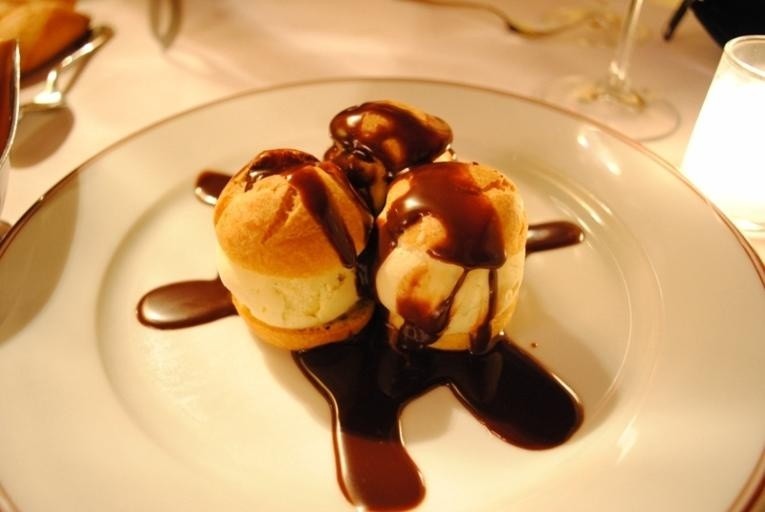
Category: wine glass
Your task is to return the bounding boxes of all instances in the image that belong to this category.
[0,2,21,255]
[551,0,682,141]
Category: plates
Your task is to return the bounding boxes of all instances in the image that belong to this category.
[3,75,764,511]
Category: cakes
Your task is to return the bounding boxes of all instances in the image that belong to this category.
[1,0,93,76]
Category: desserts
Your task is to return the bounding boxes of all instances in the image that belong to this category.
[210,98,530,355]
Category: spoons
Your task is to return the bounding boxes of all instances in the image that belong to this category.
[15,24,113,113]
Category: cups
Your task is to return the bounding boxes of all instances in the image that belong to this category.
[680,37,765,247]
